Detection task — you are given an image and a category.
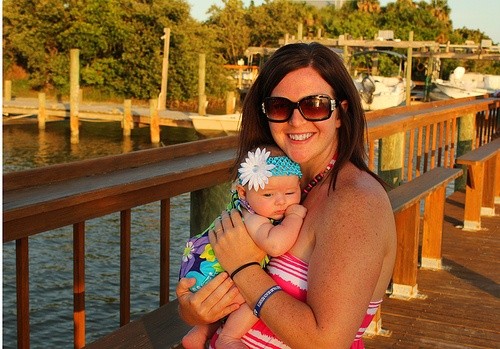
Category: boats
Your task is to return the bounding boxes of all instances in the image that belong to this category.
[344,49,416,111]
[431,78,488,100]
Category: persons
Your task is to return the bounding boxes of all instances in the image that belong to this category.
[179,144,309,349]
[175,41,398,349]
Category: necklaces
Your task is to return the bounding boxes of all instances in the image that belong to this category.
[299,152,339,204]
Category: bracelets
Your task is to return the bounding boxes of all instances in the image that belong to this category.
[230,261,261,280]
[253,285,283,318]
